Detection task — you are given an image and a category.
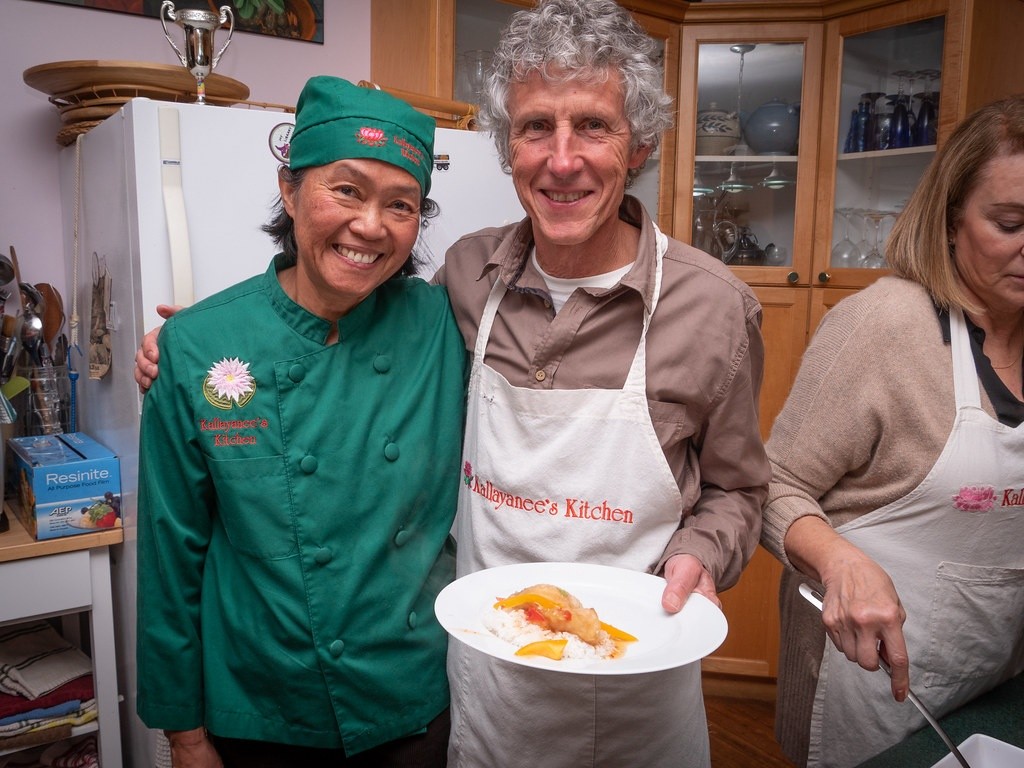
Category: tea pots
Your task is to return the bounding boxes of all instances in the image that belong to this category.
[722,234,763,267]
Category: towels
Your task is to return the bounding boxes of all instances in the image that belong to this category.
[0,616,101,768]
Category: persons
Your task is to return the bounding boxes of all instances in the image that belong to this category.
[762,92,1024,767]
[132,74,474,767]
[133,0,775,768]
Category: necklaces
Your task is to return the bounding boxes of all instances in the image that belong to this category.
[992,347,1023,369]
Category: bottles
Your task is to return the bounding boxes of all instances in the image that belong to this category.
[844,100,932,153]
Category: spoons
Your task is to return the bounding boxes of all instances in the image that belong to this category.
[22,315,48,389]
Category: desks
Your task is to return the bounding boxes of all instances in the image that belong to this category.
[0,499,128,768]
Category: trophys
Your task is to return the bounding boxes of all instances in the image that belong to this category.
[158,1,235,106]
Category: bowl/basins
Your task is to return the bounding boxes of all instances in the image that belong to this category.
[930,733,1024,768]
[209,0,316,41]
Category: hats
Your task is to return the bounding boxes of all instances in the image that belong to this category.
[289,76,436,199]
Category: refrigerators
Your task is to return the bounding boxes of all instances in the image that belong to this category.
[59,97,525,768]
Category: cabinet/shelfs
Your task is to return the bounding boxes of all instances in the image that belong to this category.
[670,0,1024,704]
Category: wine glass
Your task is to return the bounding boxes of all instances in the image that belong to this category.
[862,68,941,152]
[465,50,492,103]
[830,206,906,268]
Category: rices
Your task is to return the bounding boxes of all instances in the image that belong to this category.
[481,598,603,660]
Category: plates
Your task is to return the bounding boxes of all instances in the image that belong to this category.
[67,506,98,530]
[434,562,728,675]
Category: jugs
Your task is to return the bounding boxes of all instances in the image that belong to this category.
[694,209,739,264]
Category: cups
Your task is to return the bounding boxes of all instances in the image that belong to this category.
[766,247,786,267]
[21,369,73,436]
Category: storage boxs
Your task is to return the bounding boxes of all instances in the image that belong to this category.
[3,435,124,538]
